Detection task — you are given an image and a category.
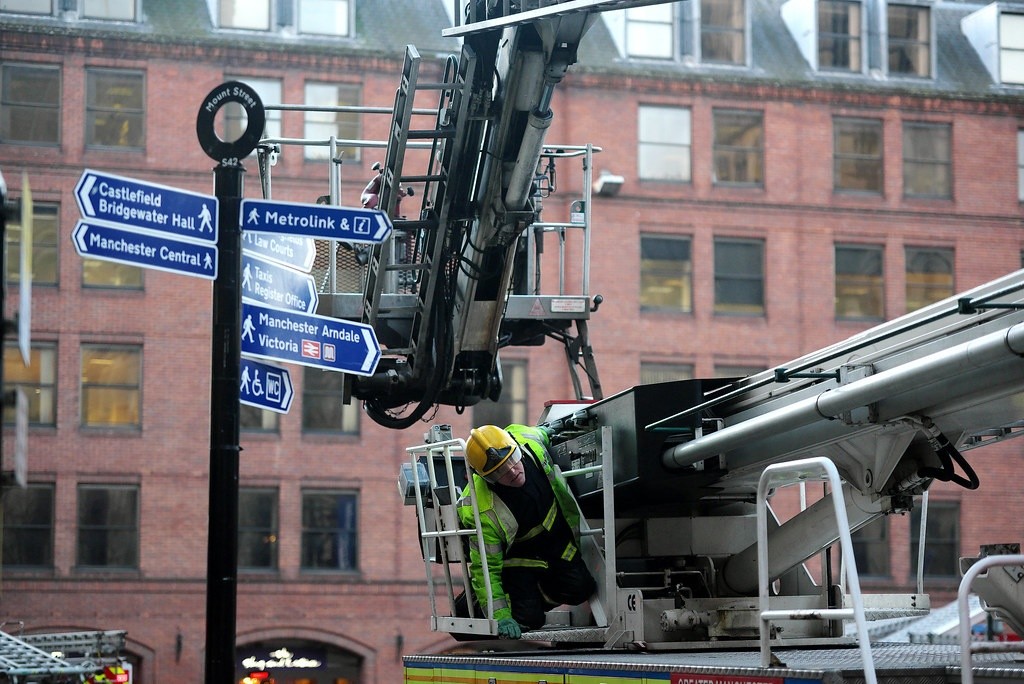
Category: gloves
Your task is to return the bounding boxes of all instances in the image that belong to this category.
[496,617,531,639]
[538,426,556,436]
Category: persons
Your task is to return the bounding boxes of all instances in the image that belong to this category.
[450,424,594,642]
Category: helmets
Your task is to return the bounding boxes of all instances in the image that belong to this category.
[464,424,519,480]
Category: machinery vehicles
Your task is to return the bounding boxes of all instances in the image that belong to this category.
[252,0,1024,683]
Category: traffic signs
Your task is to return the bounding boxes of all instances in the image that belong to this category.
[71,167,393,414]
